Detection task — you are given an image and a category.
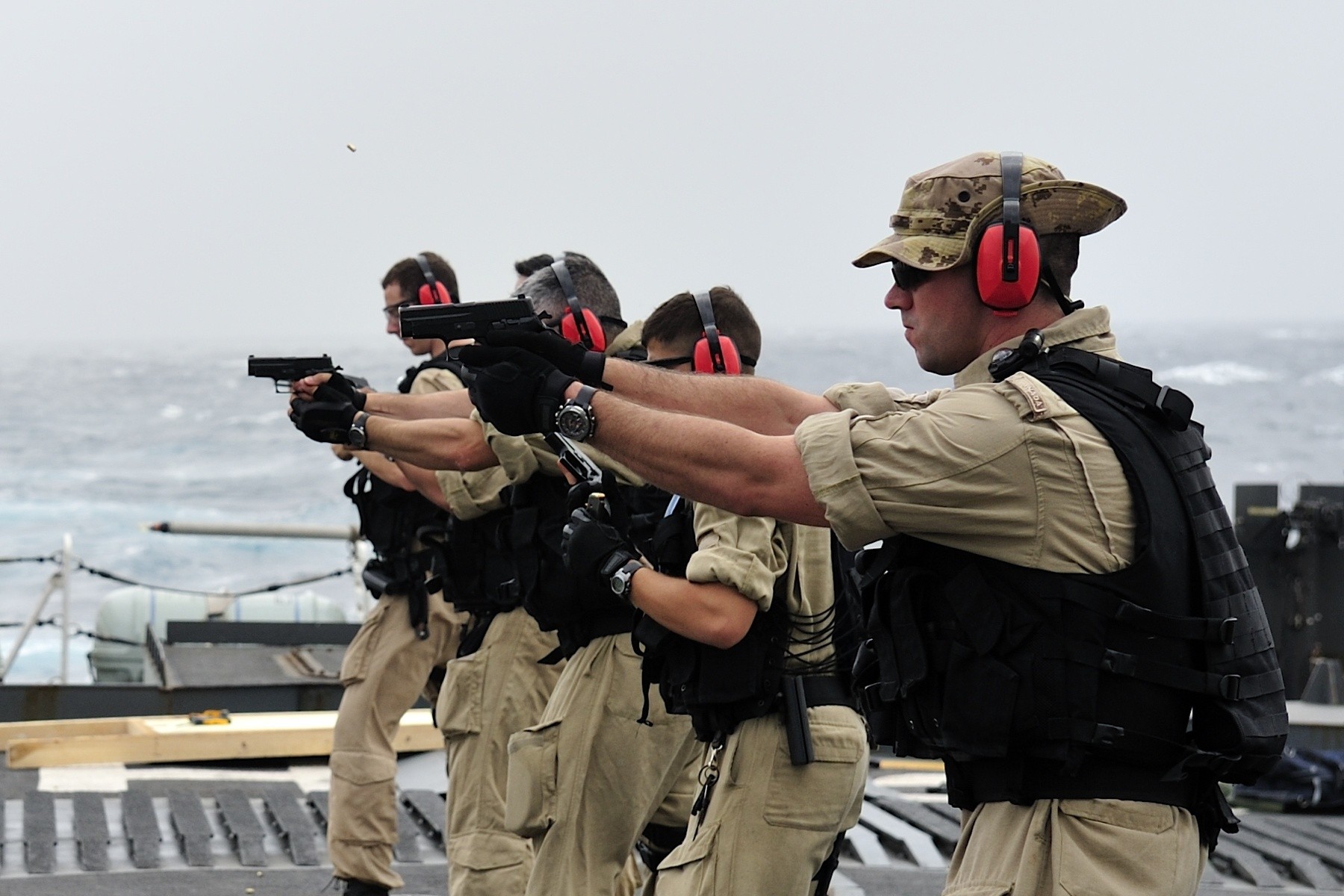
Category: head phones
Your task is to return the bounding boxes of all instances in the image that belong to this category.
[972,149,1043,317]
[550,259,607,353]
[413,254,452,304]
[690,291,742,374]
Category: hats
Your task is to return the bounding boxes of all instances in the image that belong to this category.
[851,151,1128,272]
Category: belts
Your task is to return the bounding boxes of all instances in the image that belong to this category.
[734,674,863,722]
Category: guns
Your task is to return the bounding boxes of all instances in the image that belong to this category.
[249,353,342,394]
[541,429,618,519]
[395,293,554,353]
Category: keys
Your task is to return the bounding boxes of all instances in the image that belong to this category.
[690,764,721,841]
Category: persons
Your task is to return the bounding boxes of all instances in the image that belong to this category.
[454,153,1289,894]
[286,252,715,895]
[558,283,872,896]
[327,250,477,894]
[339,253,591,657]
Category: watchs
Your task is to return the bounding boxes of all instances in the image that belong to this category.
[555,383,602,444]
[610,561,644,604]
[347,413,375,448]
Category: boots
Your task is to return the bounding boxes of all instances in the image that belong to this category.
[334,876,390,896]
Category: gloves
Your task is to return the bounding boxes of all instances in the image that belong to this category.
[457,344,581,437]
[560,508,642,584]
[472,322,605,391]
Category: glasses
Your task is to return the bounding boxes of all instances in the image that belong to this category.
[384,299,417,321]
[891,259,934,291]
